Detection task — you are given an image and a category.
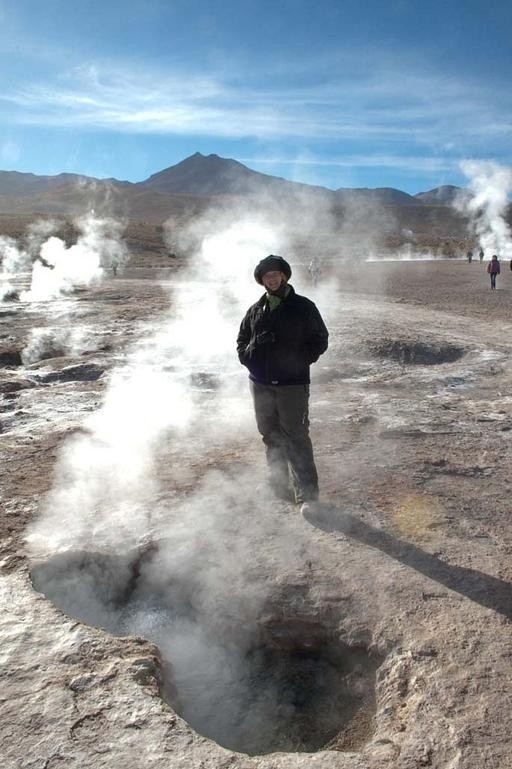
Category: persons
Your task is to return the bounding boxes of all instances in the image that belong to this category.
[487,255,500,290]
[466,250,473,263]
[479,250,484,263]
[236,255,329,516]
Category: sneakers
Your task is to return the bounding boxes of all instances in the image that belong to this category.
[301,502,311,513]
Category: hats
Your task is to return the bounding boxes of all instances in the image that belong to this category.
[254,255,292,286]
